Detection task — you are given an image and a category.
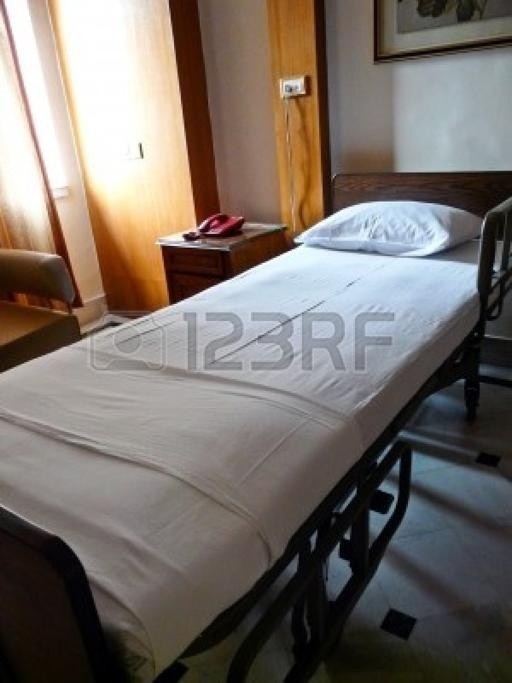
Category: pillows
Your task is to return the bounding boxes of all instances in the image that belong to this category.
[291,199,483,259]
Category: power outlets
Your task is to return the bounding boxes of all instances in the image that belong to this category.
[278,75,308,98]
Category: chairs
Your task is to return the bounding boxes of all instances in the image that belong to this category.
[0,248,84,373]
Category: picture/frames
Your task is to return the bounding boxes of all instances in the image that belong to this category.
[371,0,512,64]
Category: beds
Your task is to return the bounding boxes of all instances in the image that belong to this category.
[0,170,512,681]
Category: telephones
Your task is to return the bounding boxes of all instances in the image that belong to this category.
[196,212,245,237]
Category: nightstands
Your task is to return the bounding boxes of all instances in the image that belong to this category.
[155,221,289,305]
